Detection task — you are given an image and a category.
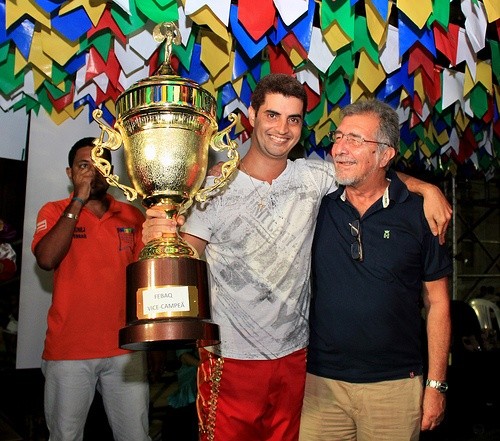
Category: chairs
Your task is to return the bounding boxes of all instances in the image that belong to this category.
[449,296,500,416]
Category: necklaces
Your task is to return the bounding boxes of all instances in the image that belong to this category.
[242,160,284,207]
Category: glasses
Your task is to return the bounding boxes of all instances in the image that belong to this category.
[327,131,390,147]
[349,220,362,260]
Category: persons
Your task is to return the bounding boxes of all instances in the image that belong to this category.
[208,74,451,441]
[0,219,22,441]
[142,74,453,441]
[31,137,152,441]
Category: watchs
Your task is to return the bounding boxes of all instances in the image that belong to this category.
[426,379,448,393]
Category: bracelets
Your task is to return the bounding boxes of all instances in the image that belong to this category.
[73,197,84,205]
[62,212,78,219]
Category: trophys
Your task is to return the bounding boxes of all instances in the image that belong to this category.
[91,20,240,350]
